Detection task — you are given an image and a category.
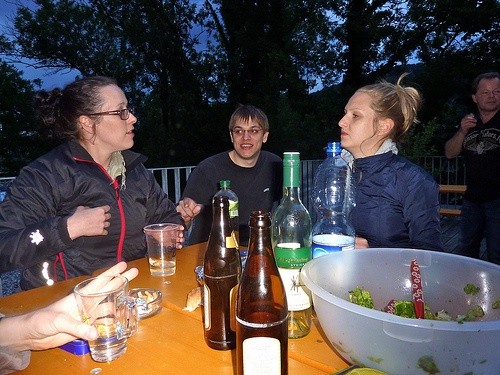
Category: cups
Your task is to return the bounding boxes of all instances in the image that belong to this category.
[73,274,128,364]
[144,224,179,276]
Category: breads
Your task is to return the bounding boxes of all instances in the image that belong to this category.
[186,287,205,312]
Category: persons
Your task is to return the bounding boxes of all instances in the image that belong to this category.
[176,105,283,246]
[0,261,139,375]
[443,72,500,267]
[334,71,445,254]
[0,76,187,290]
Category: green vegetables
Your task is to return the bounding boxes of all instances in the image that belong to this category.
[350,283,500,375]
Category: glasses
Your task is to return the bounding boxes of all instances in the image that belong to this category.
[89,108,132,121]
[232,128,265,135]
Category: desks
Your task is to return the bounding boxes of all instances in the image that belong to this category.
[0,236,358,375]
[439,183,466,196]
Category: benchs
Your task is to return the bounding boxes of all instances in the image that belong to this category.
[439,208,461,230]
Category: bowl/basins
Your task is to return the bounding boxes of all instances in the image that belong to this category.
[300,246,500,375]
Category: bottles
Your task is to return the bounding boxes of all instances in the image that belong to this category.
[202,195,242,350]
[237,209,289,375]
[311,142,356,258]
[272,150,312,339]
[212,178,238,249]
